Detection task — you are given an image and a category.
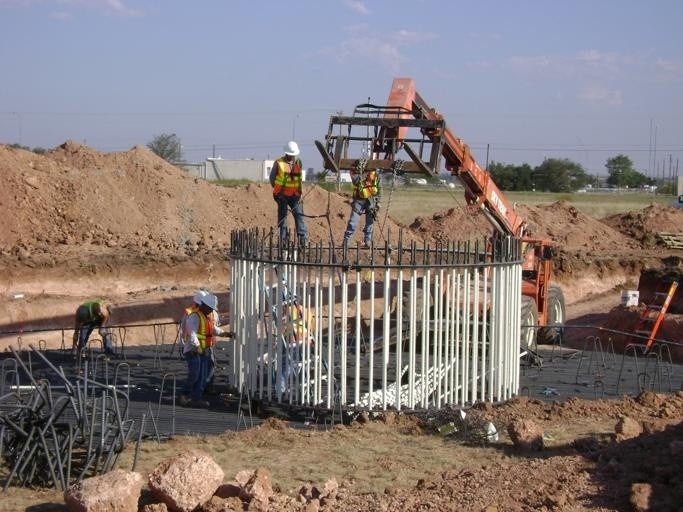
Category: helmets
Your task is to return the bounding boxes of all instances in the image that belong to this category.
[199,294,220,312]
[284,140,300,157]
[192,290,209,306]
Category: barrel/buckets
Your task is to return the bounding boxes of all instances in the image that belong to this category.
[621,290,640,307]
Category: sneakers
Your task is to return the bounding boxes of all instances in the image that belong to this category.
[340,238,372,249]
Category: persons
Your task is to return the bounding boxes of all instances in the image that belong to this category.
[69,298,114,358]
[268,140,310,247]
[178,294,235,407]
[178,290,219,383]
[270,286,315,401]
[339,160,383,248]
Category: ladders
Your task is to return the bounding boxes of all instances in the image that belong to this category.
[624,281,678,357]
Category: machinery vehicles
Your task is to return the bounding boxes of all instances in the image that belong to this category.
[605,255,683,355]
[312,74,567,361]
